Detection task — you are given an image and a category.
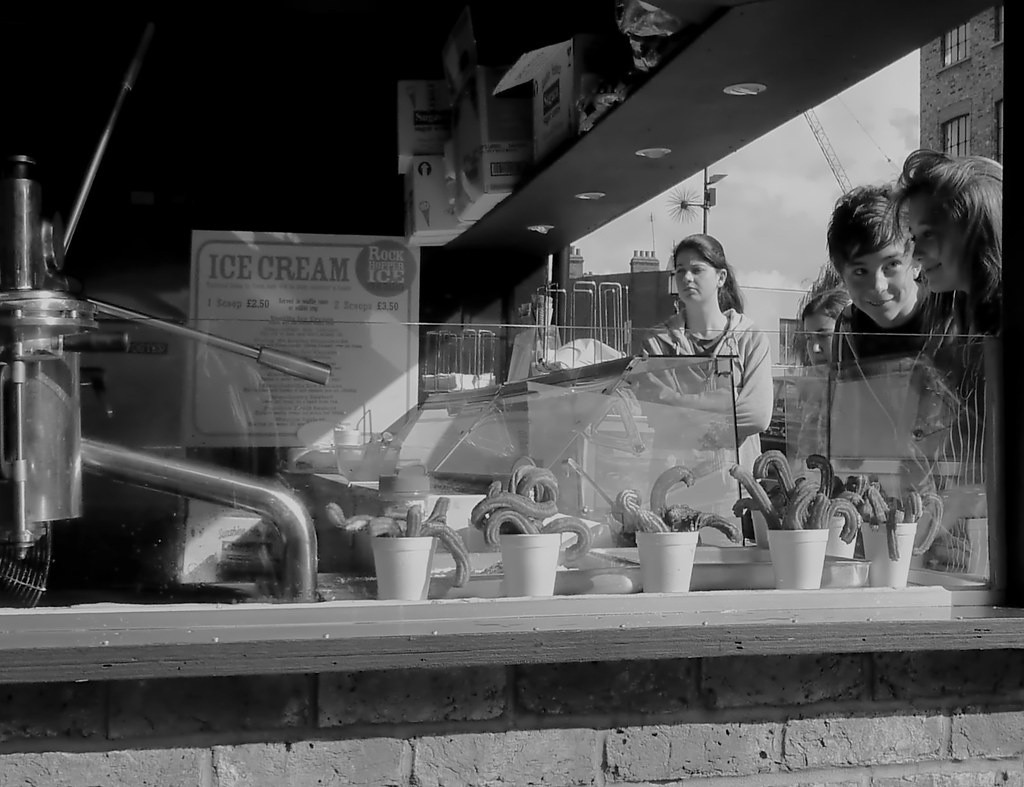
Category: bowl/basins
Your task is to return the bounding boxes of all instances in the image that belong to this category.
[333,445,401,483]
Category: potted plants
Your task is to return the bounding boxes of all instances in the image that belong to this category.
[324,452,944,600]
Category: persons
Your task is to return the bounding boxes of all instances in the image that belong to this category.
[792,149,1002,584]
[641,234,773,523]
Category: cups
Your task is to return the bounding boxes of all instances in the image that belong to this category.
[333,428,361,475]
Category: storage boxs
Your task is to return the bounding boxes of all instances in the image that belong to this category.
[395,35,606,246]
